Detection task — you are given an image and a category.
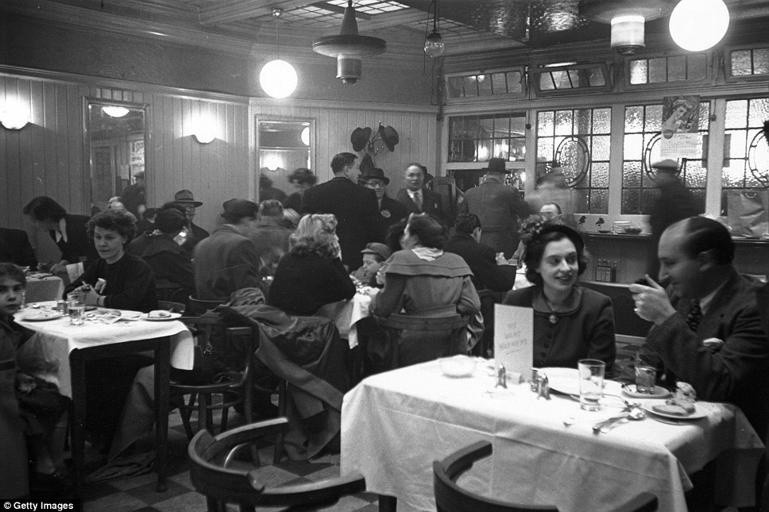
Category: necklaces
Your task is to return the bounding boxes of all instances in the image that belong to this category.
[542,291,575,325]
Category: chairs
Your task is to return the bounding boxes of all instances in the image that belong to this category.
[185,417,400,511]
[431,441,658,511]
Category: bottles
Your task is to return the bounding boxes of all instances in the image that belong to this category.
[592,256,620,283]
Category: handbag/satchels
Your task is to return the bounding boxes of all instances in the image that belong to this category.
[174,321,245,383]
[364,331,392,370]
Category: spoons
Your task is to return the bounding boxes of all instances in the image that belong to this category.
[593,406,647,434]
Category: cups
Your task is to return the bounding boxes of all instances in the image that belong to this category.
[576,359,606,412]
[66,293,83,325]
[633,363,656,391]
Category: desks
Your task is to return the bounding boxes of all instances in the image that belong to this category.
[10,299,196,512]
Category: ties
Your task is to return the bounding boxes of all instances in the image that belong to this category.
[685,304,703,332]
[414,193,422,212]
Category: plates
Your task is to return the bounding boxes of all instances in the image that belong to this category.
[623,384,670,401]
[440,350,610,406]
[639,396,708,422]
[20,296,134,330]
[141,310,186,323]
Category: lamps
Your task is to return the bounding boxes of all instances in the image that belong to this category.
[301,127,309,148]
[101,106,130,118]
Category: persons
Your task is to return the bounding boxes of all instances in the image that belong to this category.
[645,158,699,279]
[191,197,278,418]
[23,196,99,284]
[61,210,160,476]
[465,160,534,260]
[528,161,579,223]
[538,201,566,224]
[247,152,441,287]
[93,190,209,305]
[120,171,145,211]
[0,227,38,270]
[502,224,616,379]
[269,212,356,317]
[365,212,485,366]
[0,261,71,500]
[628,215,769,445]
[445,214,515,290]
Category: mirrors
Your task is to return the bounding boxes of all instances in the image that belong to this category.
[255,113,317,205]
[84,95,150,218]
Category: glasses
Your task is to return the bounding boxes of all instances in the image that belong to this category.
[367,182,385,187]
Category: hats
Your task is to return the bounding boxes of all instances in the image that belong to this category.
[351,127,371,151]
[174,190,203,207]
[224,198,258,216]
[482,159,511,174]
[134,172,144,178]
[380,126,399,152]
[653,160,677,170]
[361,241,391,260]
[361,168,389,185]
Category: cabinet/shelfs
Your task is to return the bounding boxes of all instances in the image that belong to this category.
[578,233,769,338]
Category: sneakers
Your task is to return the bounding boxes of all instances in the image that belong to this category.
[34,471,73,493]
